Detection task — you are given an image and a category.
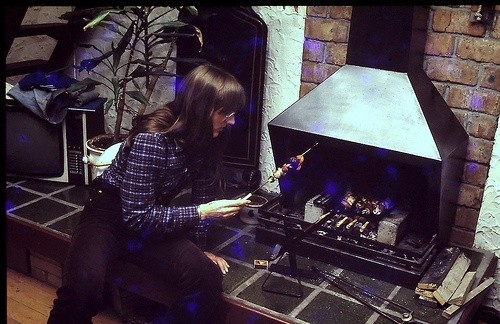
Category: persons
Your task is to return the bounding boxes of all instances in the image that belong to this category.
[46,62,251,323]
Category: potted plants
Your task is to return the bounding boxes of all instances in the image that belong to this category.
[48,4,205,185]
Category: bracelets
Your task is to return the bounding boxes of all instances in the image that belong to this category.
[196,205,201,220]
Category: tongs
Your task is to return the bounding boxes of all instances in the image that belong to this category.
[311,265,420,324]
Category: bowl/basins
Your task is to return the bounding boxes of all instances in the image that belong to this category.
[240,196,269,225]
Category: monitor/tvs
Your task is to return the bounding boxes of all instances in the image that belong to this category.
[5,94,108,187]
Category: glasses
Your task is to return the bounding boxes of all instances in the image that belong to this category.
[222,112,236,123]
